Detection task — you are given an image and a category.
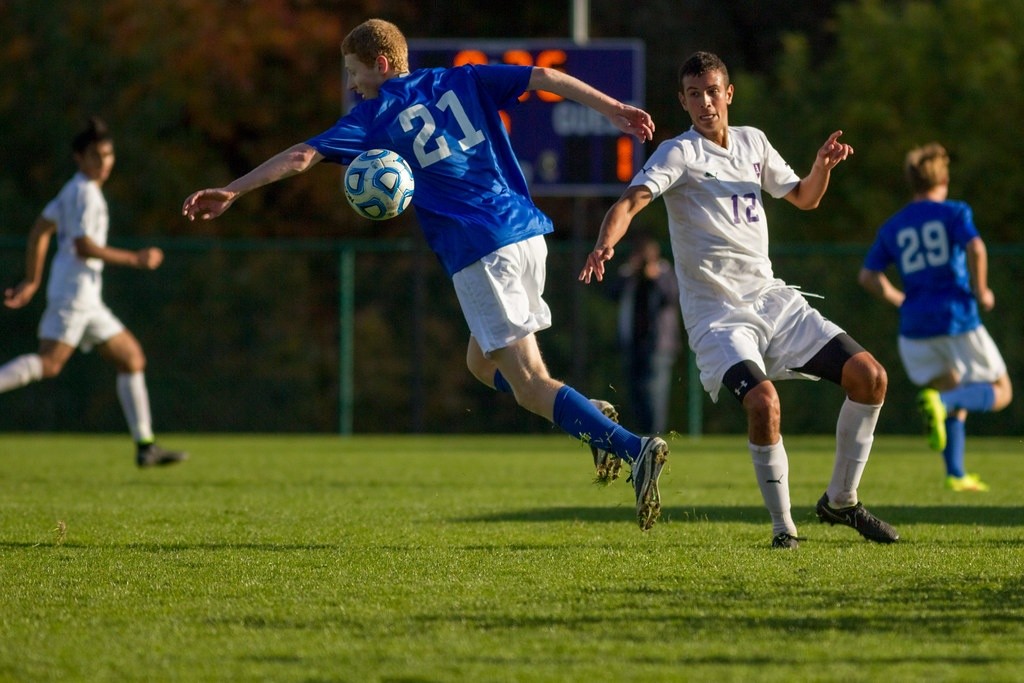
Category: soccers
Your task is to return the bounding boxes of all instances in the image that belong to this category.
[344,149,415,220]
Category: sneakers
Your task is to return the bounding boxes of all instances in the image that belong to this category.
[589,401,621,482]
[918,387,947,452]
[945,474,990,494]
[138,446,189,467]
[815,492,901,543]
[771,532,801,547]
[629,436,670,530]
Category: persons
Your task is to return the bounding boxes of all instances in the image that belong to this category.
[858,143,1015,492]
[576,49,901,549]
[1,117,190,470]
[180,18,672,533]
